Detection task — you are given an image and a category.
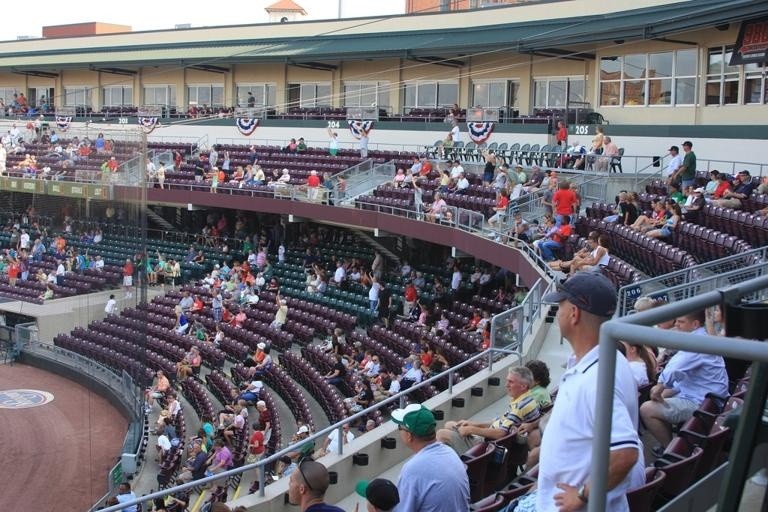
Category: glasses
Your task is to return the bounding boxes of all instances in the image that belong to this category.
[299,456,314,488]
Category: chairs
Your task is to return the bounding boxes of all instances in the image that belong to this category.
[1,140,101,362]
[100,141,766,511]
[2,105,596,125]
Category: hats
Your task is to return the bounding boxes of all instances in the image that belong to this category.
[391,403,436,436]
[257,342,266,349]
[544,271,617,318]
[355,478,400,510]
[296,426,308,435]
